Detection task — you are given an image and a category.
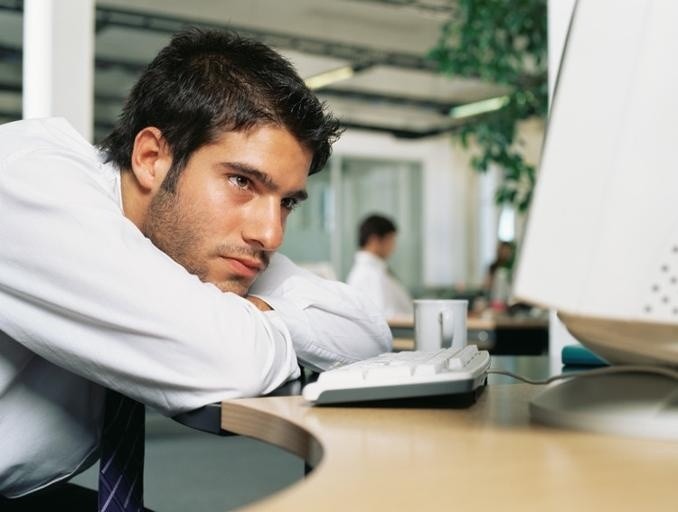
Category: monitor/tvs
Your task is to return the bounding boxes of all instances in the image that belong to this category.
[509,1,678,438]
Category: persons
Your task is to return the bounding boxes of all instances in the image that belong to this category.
[345,213,415,319]
[0,27,394,512]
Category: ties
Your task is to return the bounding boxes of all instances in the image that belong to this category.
[97,388,147,512]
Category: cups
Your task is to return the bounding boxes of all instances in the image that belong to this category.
[413,299,468,351]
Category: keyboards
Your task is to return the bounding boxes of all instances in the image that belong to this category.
[301,342,491,409]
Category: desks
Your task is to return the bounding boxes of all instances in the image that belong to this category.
[382,314,549,354]
[218,382,677,512]
